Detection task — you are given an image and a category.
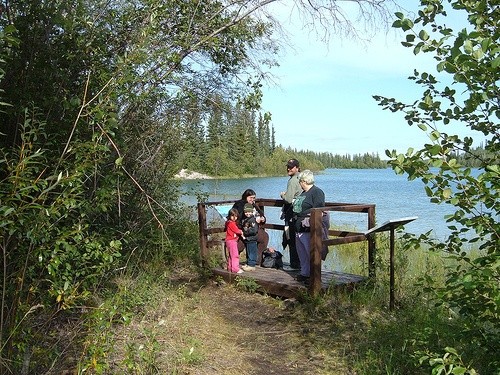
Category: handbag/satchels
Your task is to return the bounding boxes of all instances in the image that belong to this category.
[261,248,284,268]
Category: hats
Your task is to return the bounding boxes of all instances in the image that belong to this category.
[286,159,299,168]
[244,203,253,212]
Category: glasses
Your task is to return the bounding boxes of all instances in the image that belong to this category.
[297,176,304,182]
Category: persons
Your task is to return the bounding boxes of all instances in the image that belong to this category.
[225,208,244,273]
[281,159,305,271]
[232,189,269,268]
[242,203,259,271]
[291,169,329,281]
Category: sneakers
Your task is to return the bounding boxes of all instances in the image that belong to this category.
[241,264,256,271]
[294,273,310,281]
[282,264,302,271]
[236,269,244,273]
[221,261,229,270]
